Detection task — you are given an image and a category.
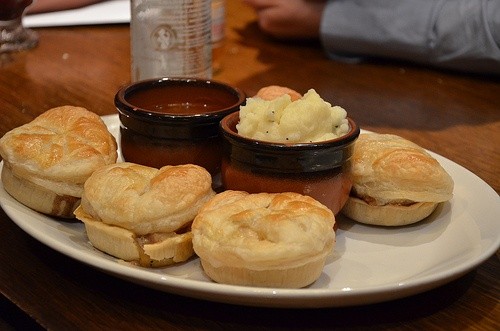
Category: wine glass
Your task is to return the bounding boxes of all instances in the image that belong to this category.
[0,0,40,53]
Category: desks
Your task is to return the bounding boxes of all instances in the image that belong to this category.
[0,1,500,331]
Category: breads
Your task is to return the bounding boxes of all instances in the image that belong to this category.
[191,190,336,289]
[73,162,217,267]
[341,135,453,226]
[257,86,301,102]
[0,105,118,218]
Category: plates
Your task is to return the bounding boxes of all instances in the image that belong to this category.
[0,115,500,309]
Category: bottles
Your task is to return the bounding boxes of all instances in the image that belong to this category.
[129,0,212,84]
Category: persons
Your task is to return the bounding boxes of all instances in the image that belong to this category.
[25,0,104,15]
[245,0,500,76]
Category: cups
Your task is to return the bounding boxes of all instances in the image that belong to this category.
[113,79,247,195]
[211,0,225,44]
[219,110,360,215]
